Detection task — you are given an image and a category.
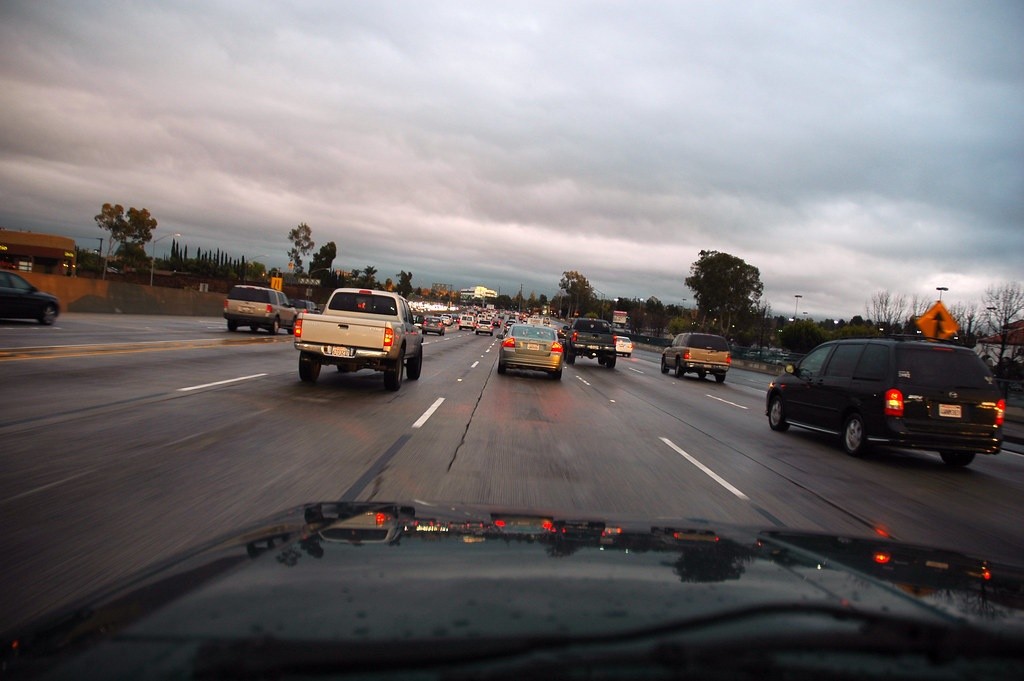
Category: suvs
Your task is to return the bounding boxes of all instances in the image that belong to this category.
[459,315,476,331]
[661,332,731,383]
[223,285,297,335]
[476,320,494,336]
[762,334,1009,467]
[564,319,618,369]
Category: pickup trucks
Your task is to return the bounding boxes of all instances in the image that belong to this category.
[292,288,425,390]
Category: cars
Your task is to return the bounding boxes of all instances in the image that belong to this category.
[0,270,61,326]
[0,260,16,270]
[615,336,633,357]
[433,309,570,341]
[412,314,425,328]
[421,317,446,336]
[496,324,565,380]
[290,300,321,315]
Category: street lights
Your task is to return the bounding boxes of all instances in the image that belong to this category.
[307,268,330,301]
[149,233,182,286]
[988,306,997,337]
[935,287,948,304]
[682,298,687,318]
[794,295,802,324]
